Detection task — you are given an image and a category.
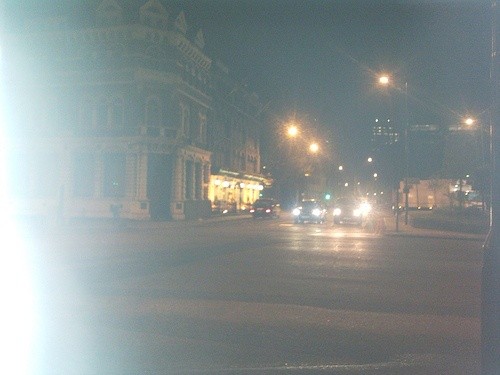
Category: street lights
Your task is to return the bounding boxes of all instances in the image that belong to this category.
[214,178,264,203]
[285,119,302,209]
[307,141,323,200]
[377,71,411,232]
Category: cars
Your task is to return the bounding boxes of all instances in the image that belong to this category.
[293,198,328,224]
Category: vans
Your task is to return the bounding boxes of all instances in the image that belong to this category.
[249,197,283,221]
[333,196,364,228]
